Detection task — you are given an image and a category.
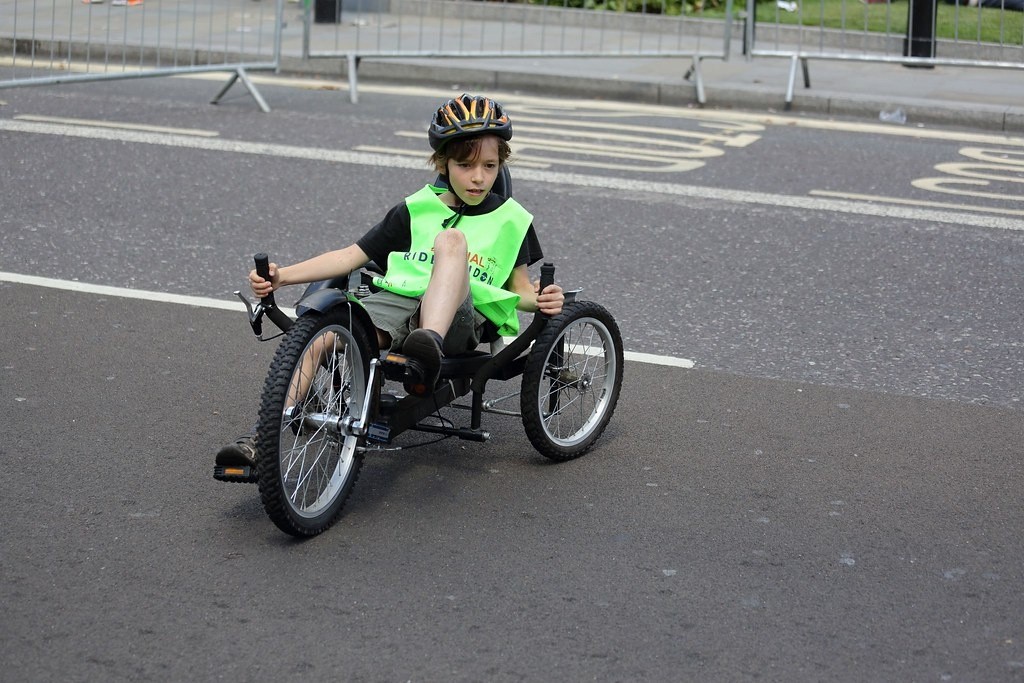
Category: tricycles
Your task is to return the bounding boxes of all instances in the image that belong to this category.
[212,163,626,541]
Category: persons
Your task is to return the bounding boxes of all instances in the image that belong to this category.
[212,94,563,484]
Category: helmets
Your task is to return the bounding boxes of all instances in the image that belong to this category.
[427,92,513,150]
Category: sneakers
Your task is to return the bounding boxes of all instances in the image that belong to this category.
[215,432,259,467]
[402,329,442,396]
